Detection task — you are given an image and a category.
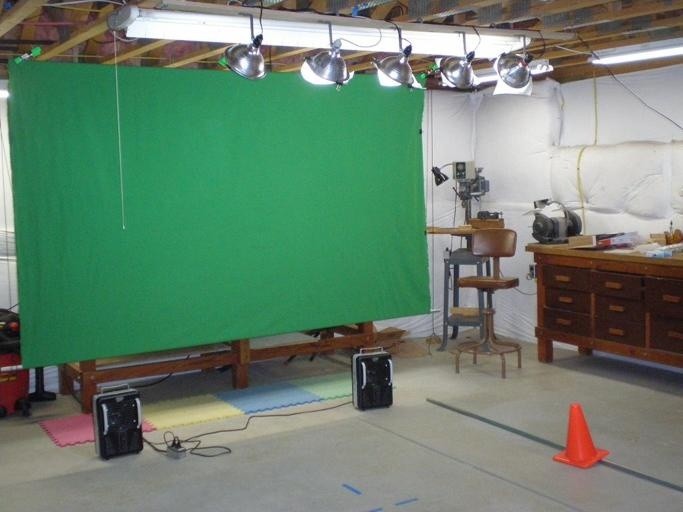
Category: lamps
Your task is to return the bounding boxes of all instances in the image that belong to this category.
[431,163,452,186]
[474,58,554,84]
[588,37,683,66]
[224,13,266,80]
[492,34,534,90]
[107,2,532,60]
[374,21,417,90]
[440,32,475,90]
[299,21,350,91]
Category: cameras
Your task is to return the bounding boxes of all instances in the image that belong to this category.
[453,161,489,198]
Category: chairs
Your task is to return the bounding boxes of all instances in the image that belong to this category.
[455,229,522,379]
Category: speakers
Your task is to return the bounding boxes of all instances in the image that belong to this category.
[351,346,392,410]
[92,383,143,461]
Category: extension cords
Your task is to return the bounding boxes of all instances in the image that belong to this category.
[166,441,187,458]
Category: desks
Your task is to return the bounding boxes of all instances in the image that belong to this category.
[525,242,683,369]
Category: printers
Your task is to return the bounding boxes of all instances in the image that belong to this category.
[532,198,583,245]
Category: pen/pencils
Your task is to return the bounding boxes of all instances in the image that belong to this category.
[669,219,672,233]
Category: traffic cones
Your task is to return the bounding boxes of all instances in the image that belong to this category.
[553,404,608,469]
